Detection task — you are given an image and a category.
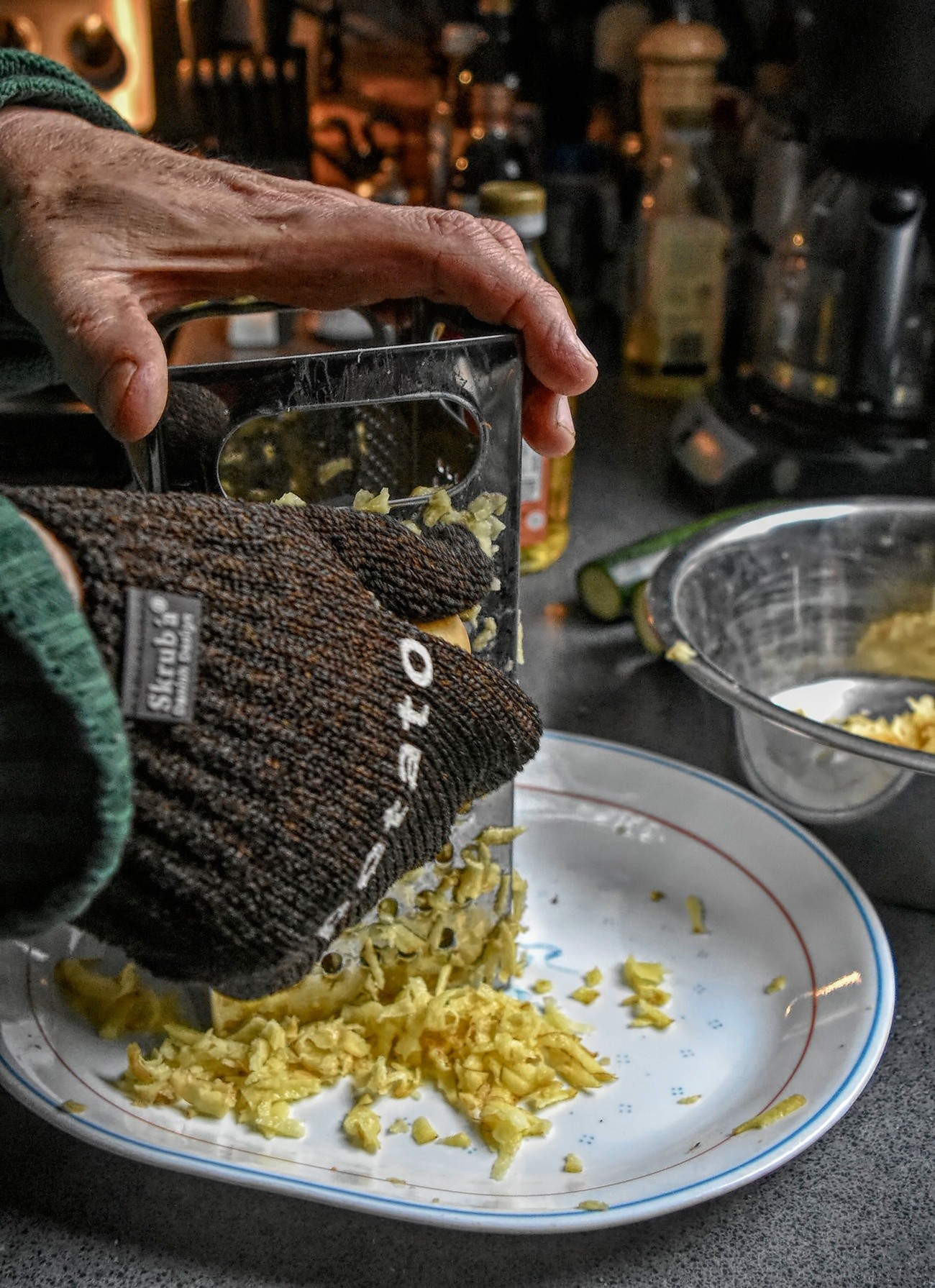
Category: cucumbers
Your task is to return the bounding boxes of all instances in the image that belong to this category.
[575,498,778,657]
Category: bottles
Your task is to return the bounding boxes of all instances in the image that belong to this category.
[474,181,573,573]
[303,0,924,413]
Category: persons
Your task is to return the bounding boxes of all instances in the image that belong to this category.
[0,45,600,999]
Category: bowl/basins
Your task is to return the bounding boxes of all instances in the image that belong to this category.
[647,493,934,915]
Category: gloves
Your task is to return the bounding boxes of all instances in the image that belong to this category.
[3,478,547,1007]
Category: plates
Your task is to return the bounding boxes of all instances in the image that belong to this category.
[0,729,896,1237]
